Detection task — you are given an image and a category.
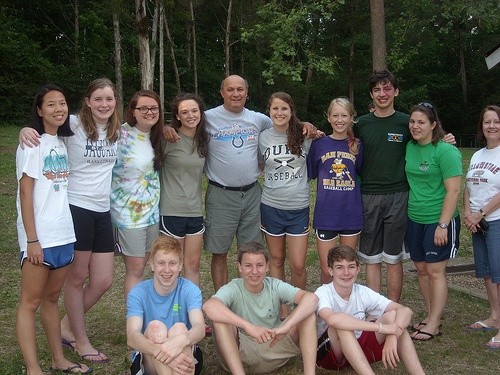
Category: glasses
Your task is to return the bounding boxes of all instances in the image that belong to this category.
[135,106,160,114]
[417,102,432,108]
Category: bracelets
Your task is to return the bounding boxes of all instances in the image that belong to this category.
[378,323,383,333]
[479,209,486,218]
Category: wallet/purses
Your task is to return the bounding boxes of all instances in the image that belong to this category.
[475,218,489,238]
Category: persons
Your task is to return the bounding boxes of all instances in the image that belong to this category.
[204,243,319,375]
[306,98,364,286]
[158,93,214,336]
[127,235,205,375]
[313,70,457,301]
[16,84,92,375]
[258,91,328,321]
[161,74,317,293]
[314,245,428,375]
[111,89,163,295]
[406,102,462,340]
[20,78,120,363]
[460,106,500,350]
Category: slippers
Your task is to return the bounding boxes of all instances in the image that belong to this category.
[485,335,500,350]
[75,346,109,363]
[50,359,93,375]
[466,320,500,331]
[410,327,444,341]
[411,317,445,332]
[61,337,76,351]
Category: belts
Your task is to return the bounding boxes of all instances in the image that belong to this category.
[209,179,258,192]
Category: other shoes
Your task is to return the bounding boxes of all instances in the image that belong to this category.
[205,323,213,336]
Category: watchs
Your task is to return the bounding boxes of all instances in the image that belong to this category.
[438,223,448,229]
